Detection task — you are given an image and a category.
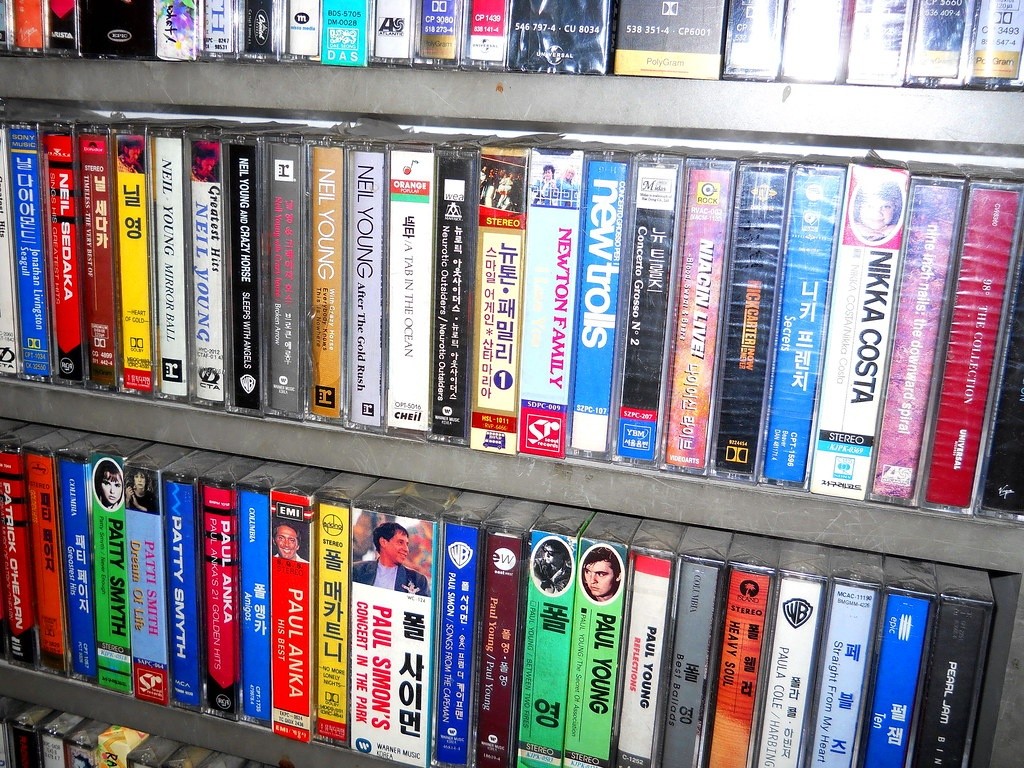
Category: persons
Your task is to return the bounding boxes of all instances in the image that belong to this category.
[118,136,144,174]
[853,182,903,241]
[584,548,621,602]
[272,520,309,564]
[98,466,123,510]
[534,540,570,594]
[353,522,432,597]
[480,166,524,212]
[532,164,578,207]
[191,141,220,182]
[125,469,158,513]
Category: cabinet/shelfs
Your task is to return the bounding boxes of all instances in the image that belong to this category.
[0,54,1024,768]
